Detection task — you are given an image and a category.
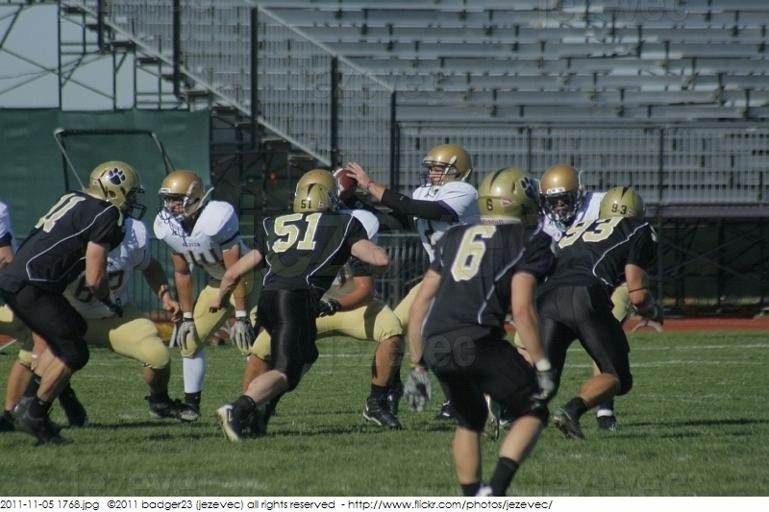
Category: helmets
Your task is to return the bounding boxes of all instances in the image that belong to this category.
[89,160,147,221]
[478,164,587,228]
[599,186,648,222]
[418,144,471,189]
[156,169,205,224]
[294,168,345,214]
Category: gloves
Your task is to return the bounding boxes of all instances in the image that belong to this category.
[633,304,665,328]
[176,317,198,351]
[229,316,257,351]
[403,363,431,412]
[318,299,342,319]
[529,364,560,404]
[101,288,123,319]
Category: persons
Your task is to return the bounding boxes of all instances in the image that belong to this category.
[0,141,662,496]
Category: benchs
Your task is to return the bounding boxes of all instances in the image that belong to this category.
[103,0,769,218]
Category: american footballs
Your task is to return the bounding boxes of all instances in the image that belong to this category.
[333,166,357,200]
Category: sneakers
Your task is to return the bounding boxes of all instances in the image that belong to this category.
[216,398,272,444]
[148,397,197,423]
[363,381,403,431]
[433,400,455,420]
[0,386,89,445]
[552,406,586,444]
[595,408,617,434]
